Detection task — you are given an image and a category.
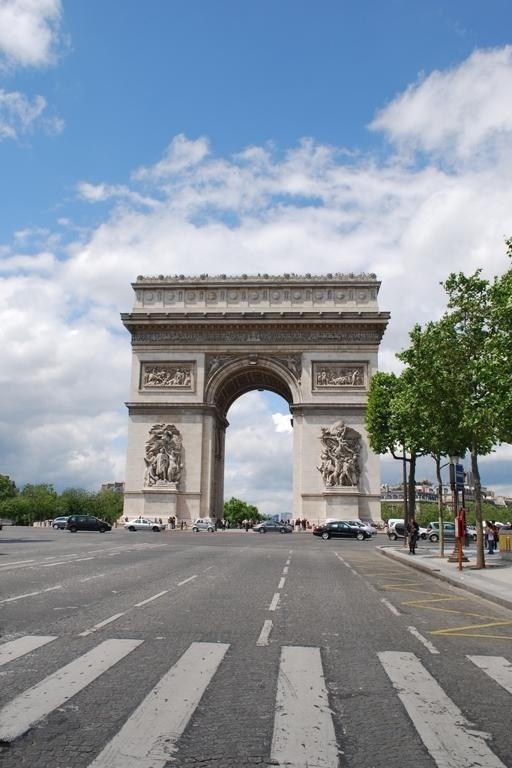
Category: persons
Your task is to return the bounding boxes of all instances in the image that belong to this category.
[124,512,311,533]
[316,421,363,486]
[367,521,389,530]
[407,518,419,555]
[484,519,500,554]
[143,364,191,386]
[144,423,184,486]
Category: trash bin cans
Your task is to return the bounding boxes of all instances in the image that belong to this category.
[498,526,512,551]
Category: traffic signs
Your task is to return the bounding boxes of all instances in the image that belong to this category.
[455,483,465,492]
[454,464,466,485]
